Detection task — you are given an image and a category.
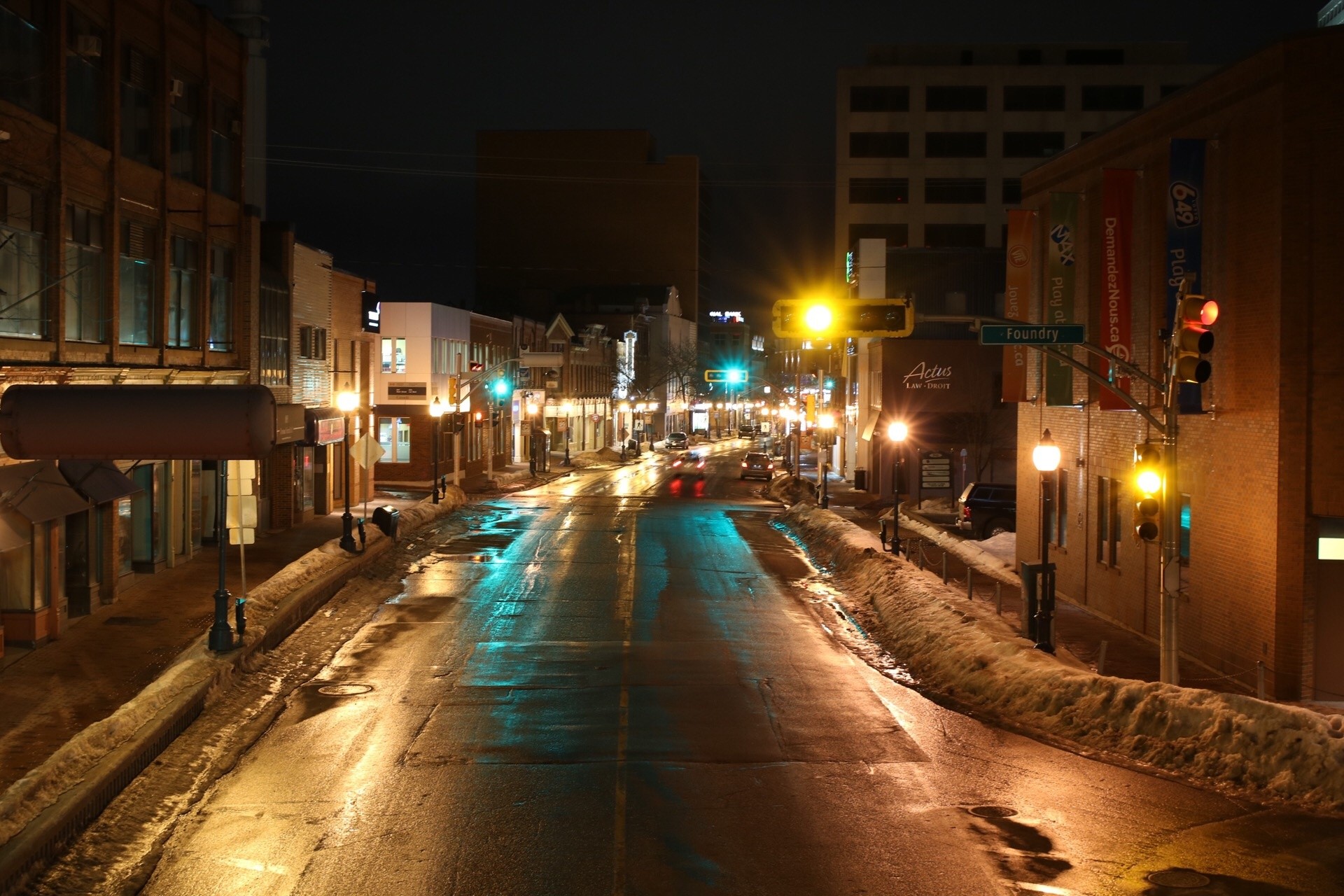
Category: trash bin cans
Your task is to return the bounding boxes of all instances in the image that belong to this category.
[853,466,866,489]
[371,504,400,546]
[627,439,637,451]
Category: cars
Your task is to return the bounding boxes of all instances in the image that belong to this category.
[666,432,690,450]
[673,452,706,479]
[740,450,773,481]
[740,423,772,439]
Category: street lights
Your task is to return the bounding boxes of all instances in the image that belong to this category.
[819,412,834,509]
[563,400,574,467]
[888,419,908,556]
[336,381,360,551]
[636,401,645,456]
[1032,427,1064,656]
[648,401,658,450]
[763,401,794,473]
[683,400,762,439]
[428,395,443,505]
[619,402,628,460]
[528,398,538,472]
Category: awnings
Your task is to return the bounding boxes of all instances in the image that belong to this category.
[60,456,146,507]
[10,465,95,526]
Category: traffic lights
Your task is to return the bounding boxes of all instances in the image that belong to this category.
[498,369,506,407]
[773,299,915,339]
[1132,444,1163,542]
[493,412,500,427]
[469,360,484,372]
[454,413,465,435]
[449,378,457,404]
[0,384,277,459]
[475,411,485,428]
[441,413,453,434]
[1176,295,1221,387]
[705,369,749,383]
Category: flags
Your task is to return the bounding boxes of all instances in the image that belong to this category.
[1045,187,1080,408]
[1163,135,1209,416]
[1098,167,1140,413]
[999,208,1040,403]
